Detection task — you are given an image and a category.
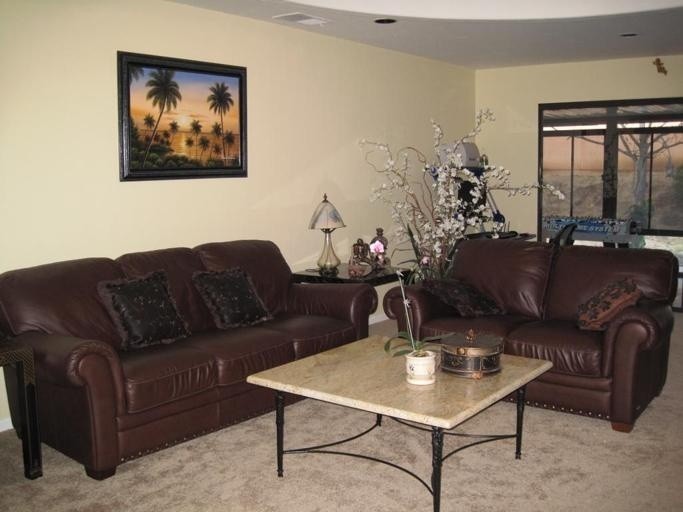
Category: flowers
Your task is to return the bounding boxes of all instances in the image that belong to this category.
[358,108,564,281]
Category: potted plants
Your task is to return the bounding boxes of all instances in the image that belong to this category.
[383,330,453,385]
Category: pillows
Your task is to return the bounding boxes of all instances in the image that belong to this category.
[96,269,191,350]
[420,278,505,319]
[573,279,643,330]
[193,267,273,329]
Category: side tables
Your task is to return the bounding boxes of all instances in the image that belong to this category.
[294,261,415,288]
[0,337,42,480]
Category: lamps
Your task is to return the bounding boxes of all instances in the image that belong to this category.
[307,192,346,274]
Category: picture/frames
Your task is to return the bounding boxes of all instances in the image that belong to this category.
[117,51,247,181]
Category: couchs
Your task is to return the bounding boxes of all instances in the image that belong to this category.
[382,238,679,432]
[0,240,377,482]
